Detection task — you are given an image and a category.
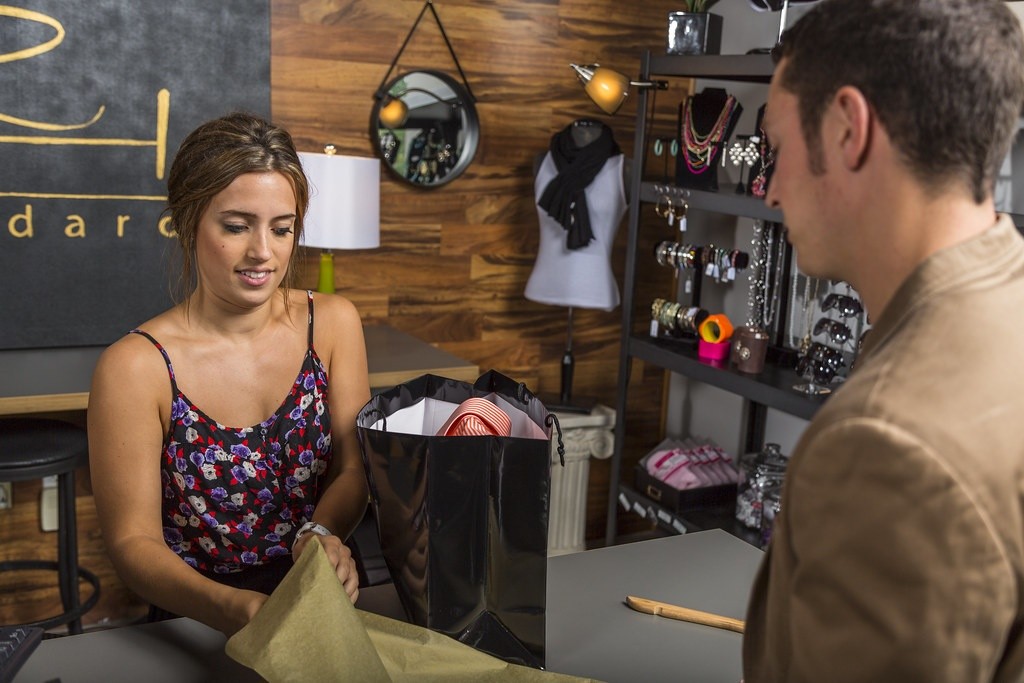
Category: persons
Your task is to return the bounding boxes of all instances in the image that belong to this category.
[524,120,630,312]
[87,112,374,639]
[674,86,742,190]
[741,0,1024,683]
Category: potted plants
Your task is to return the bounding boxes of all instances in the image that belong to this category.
[667,0,724,56]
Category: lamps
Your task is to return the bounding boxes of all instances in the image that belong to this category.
[571,62,668,114]
[380,87,455,127]
[292,144,381,296]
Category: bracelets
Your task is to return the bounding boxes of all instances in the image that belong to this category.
[292,521,331,548]
[650,195,740,336]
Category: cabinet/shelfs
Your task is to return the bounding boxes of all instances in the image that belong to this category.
[602,43,1024,557]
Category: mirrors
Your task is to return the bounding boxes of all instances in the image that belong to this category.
[369,68,481,188]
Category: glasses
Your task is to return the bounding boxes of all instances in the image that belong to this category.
[821,294,864,318]
[850,311,871,371]
[813,318,854,344]
[796,340,847,385]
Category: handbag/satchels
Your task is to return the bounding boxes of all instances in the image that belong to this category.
[355,368,566,669]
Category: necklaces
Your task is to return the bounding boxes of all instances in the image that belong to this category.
[748,220,823,356]
[682,96,777,196]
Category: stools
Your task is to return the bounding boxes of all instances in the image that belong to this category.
[0,409,101,636]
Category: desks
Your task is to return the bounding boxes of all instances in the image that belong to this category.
[0,324,481,409]
[11,528,767,683]
